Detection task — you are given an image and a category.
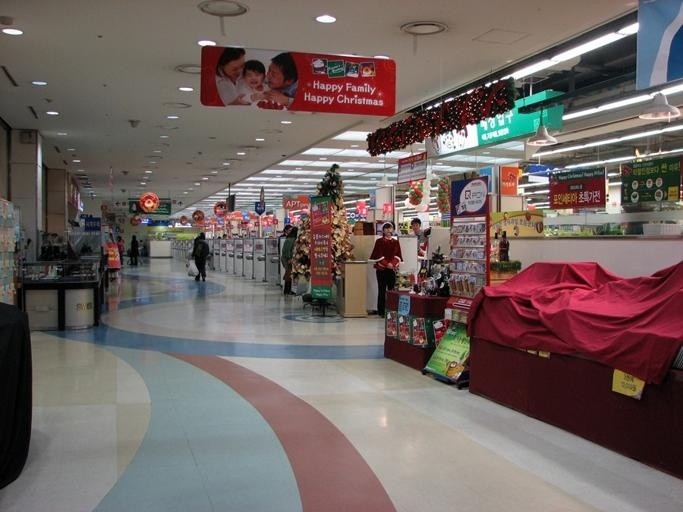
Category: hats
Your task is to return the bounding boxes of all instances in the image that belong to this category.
[381,222,393,233]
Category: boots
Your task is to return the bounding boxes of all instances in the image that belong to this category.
[284,281,296,295]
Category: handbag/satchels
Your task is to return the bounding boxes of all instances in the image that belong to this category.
[193,242,205,258]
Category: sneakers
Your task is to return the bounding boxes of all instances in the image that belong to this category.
[195,278,199,280]
[202,275,205,281]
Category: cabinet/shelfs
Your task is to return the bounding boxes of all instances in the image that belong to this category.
[384,289,447,371]
[449,224,489,299]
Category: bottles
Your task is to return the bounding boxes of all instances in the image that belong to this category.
[491,231,509,262]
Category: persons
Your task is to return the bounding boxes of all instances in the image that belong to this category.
[280,227,298,295]
[190,232,208,282]
[407,218,425,254]
[277,224,293,276]
[265,53,296,106]
[369,221,403,318]
[236,60,275,106]
[216,234,239,239]
[216,48,271,106]
[115,234,147,266]
